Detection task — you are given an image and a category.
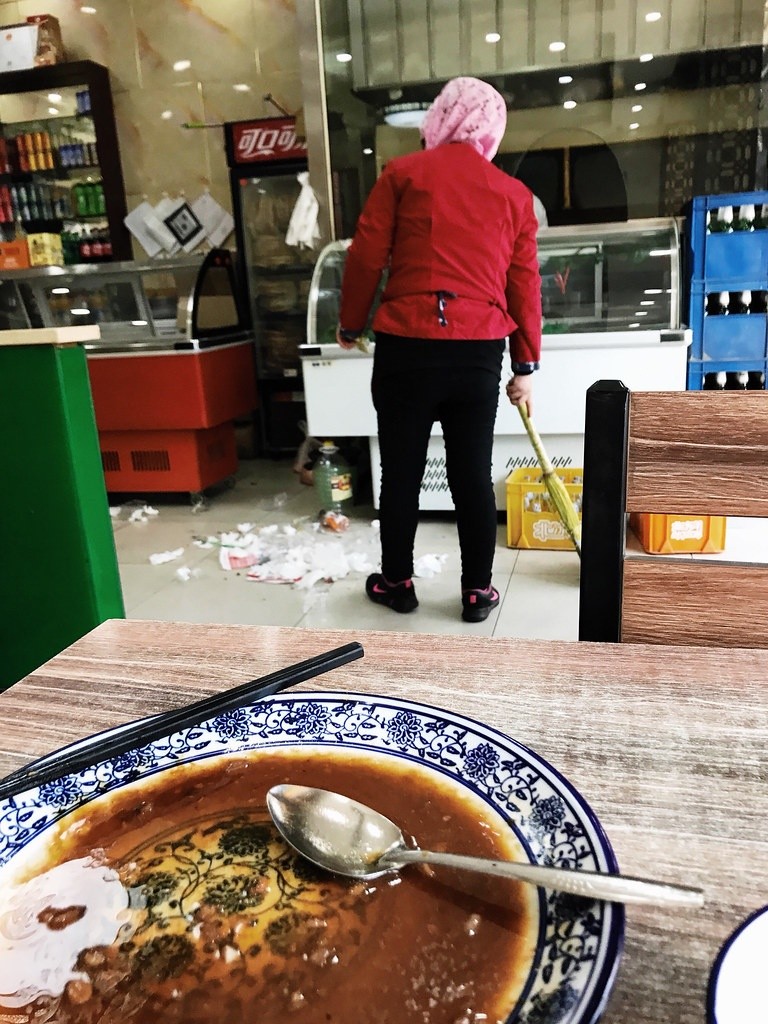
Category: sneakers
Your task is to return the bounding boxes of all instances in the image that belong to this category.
[365,572,418,614]
[462,583,499,623]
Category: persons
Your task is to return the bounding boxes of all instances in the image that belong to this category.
[336,77,541,623]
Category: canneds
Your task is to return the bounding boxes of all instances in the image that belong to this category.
[0,89,113,222]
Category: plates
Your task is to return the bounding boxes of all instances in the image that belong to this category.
[705,903,768,1024]
[0,692,627,1024]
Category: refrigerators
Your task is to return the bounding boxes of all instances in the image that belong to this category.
[224,115,316,460]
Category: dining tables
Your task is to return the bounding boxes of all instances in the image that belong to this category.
[1,620,767,1024]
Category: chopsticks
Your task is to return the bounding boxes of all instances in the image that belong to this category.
[0,642,364,804]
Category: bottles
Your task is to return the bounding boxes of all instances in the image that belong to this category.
[61,222,112,255]
[73,176,107,216]
[60,134,99,167]
[315,441,355,515]
[524,476,583,513]
[706,204,768,233]
[703,370,768,390]
[704,290,768,317]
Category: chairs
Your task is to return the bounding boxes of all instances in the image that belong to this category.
[579,380,768,650]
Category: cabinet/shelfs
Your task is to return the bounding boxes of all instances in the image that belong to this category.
[0,59,134,268]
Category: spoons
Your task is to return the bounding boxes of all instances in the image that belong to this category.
[267,785,705,909]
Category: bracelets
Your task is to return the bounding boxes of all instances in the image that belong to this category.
[511,360,540,376]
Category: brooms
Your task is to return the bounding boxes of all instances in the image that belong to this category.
[507,377,581,558]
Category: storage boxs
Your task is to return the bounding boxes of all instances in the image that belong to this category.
[629,511,727,555]
[506,466,582,552]
[685,189,768,390]
